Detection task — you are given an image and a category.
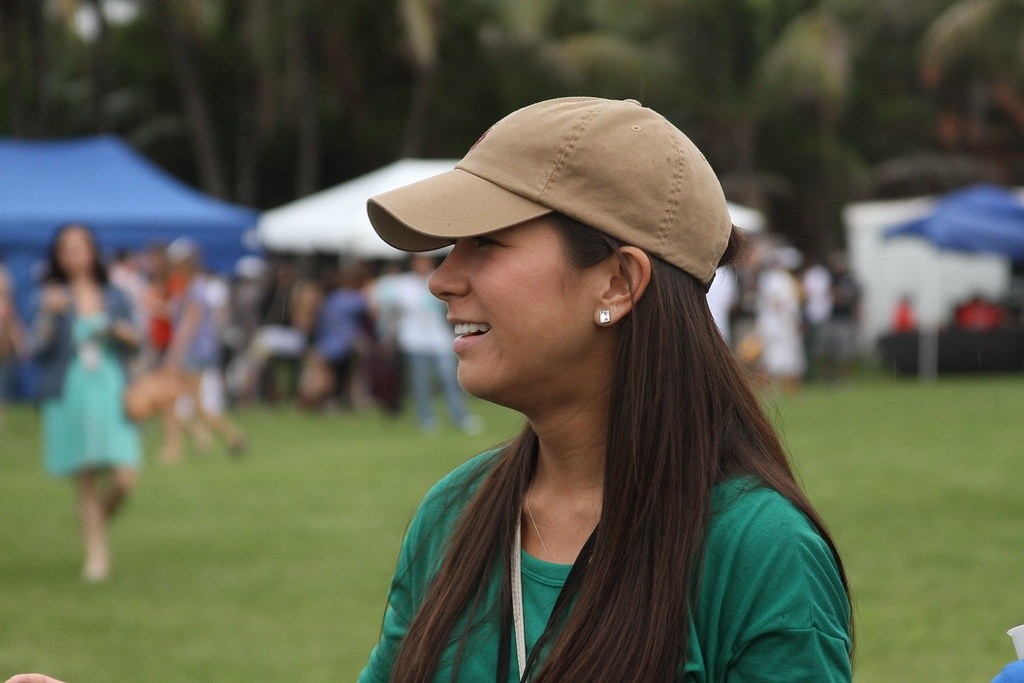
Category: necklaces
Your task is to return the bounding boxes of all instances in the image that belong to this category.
[527,471,553,560]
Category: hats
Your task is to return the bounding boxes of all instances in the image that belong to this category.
[367,95,732,293]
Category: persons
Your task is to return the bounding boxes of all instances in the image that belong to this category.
[0,220,483,582]
[705,230,1024,379]
[355,94,855,683]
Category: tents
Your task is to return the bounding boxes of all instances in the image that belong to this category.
[0,135,261,399]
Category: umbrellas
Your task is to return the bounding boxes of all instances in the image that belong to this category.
[881,183,1024,255]
[254,157,465,254]
